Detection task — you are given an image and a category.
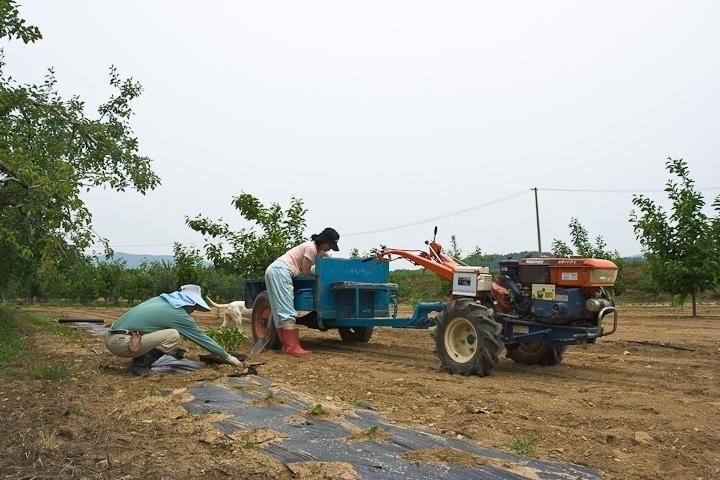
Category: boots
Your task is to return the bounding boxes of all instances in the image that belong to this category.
[130,347,167,376]
[280,327,313,356]
[275,328,284,350]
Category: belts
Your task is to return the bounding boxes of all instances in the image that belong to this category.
[110,329,132,334]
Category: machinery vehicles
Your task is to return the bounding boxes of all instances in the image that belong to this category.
[245,226,619,377]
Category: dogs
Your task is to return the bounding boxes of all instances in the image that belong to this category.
[206,294,255,339]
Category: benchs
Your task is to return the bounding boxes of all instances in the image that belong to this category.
[330,281,400,319]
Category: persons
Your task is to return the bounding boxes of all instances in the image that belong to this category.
[264,227,340,356]
[103,284,244,376]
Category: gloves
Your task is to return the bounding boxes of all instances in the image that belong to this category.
[224,354,243,368]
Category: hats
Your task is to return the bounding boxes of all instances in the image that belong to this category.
[311,227,341,252]
[180,284,212,313]
